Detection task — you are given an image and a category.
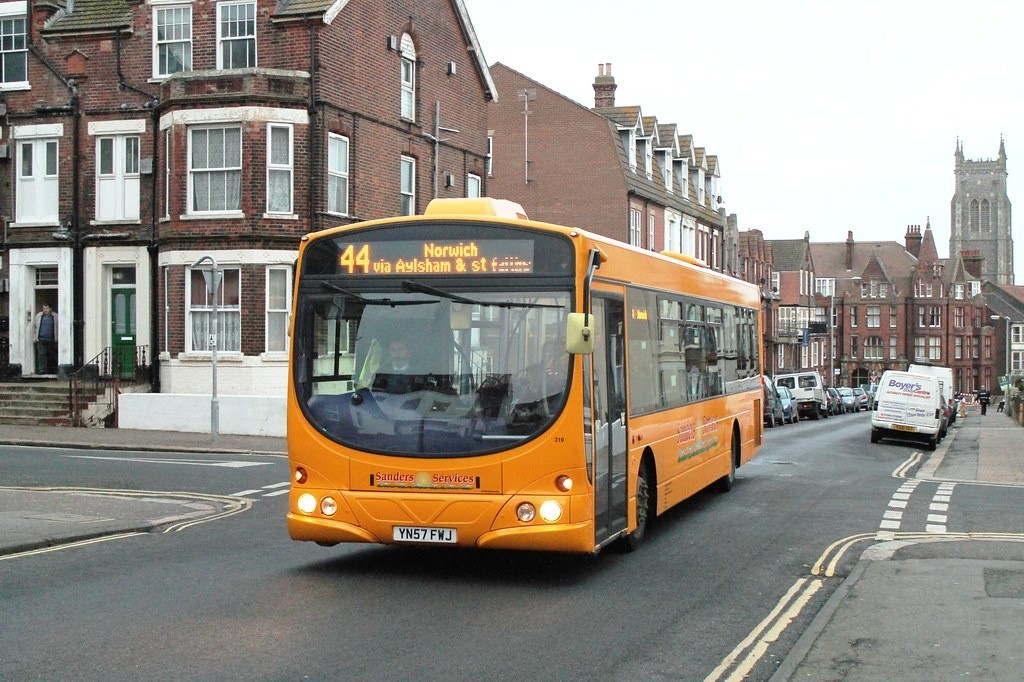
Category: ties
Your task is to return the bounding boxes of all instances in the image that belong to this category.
[395,368,399,373]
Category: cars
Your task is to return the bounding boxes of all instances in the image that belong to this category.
[837,387,861,413]
[852,388,872,411]
[940,394,950,438]
[827,388,844,415]
[775,386,800,424]
[763,374,785,428]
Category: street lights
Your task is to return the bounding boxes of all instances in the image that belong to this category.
[830,277,862,388]
[989,315,1010,374]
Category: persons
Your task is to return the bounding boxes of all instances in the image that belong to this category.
[31,303,58,374]
[975,385,990,416]
[370,337,431,394]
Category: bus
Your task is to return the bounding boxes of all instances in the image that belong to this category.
[285,195,765,555]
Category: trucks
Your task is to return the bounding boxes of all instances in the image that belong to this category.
[775,371,829,420]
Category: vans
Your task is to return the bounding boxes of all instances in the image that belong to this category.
[870,370,942,451]
[907,364,956,426]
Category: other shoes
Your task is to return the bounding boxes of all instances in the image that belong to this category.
[49,368,56,373]
[39,370,45,374]
[981,412,986,415]
